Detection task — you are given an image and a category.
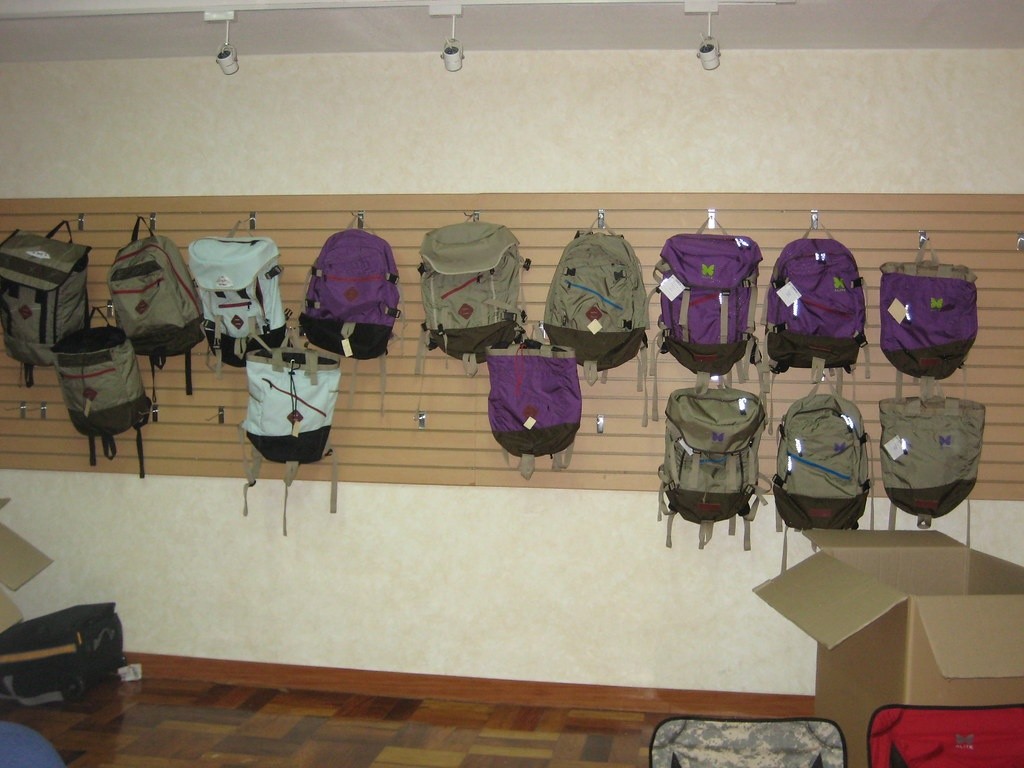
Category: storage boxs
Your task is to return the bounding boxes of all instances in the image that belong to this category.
[751,527,1024,768]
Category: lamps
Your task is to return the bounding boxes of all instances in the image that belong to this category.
[429,6,465,71]
[685,1,723,70]
[204,10,238,75]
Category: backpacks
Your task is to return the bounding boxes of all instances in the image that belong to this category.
[658,386,767,551]
[484,324,582,480]
[654,217,764,377]
[543,218,651,385]
[417,215,529,378]
[0,602,126,711]
[1,210,343,487]
[298,215,401,360]
[877,236,978,379]
[760,221,867,375]
[772,375,875,530]
[878,396,986,529]
